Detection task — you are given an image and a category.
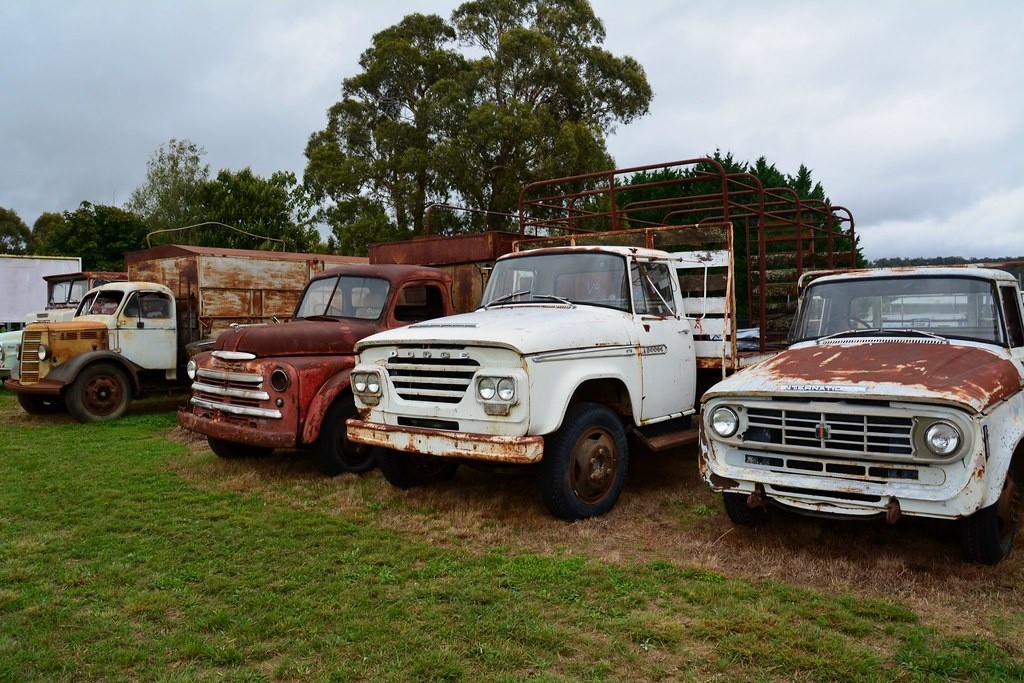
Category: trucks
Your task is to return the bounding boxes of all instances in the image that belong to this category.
[697,258,1024,567]
[175,202,581,475]
[1,271,128,383]
[2,221,369,425]
[344,157,857,522]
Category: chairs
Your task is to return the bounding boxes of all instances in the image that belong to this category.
[355,292,383,319]
